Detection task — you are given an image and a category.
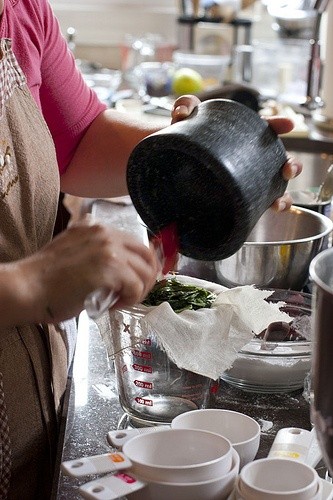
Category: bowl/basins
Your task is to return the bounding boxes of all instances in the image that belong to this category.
[241,458,318,500]
[236,473,322,500]
[78,448,240,500]
[214,206,333,289]
[126,99,290,261]
[61,428,232,483]
[219,286,312,392]
[108,409,261,471]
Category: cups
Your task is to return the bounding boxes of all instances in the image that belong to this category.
[252,37,312,104]
[124,34,158,74]
[174,49,230,91]
[109,275,234,424]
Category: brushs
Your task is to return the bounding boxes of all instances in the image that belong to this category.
[84,207,203,319]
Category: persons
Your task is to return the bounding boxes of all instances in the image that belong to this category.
[0,0,303,500]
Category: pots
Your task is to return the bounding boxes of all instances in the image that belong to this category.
[309,248,333,478]
